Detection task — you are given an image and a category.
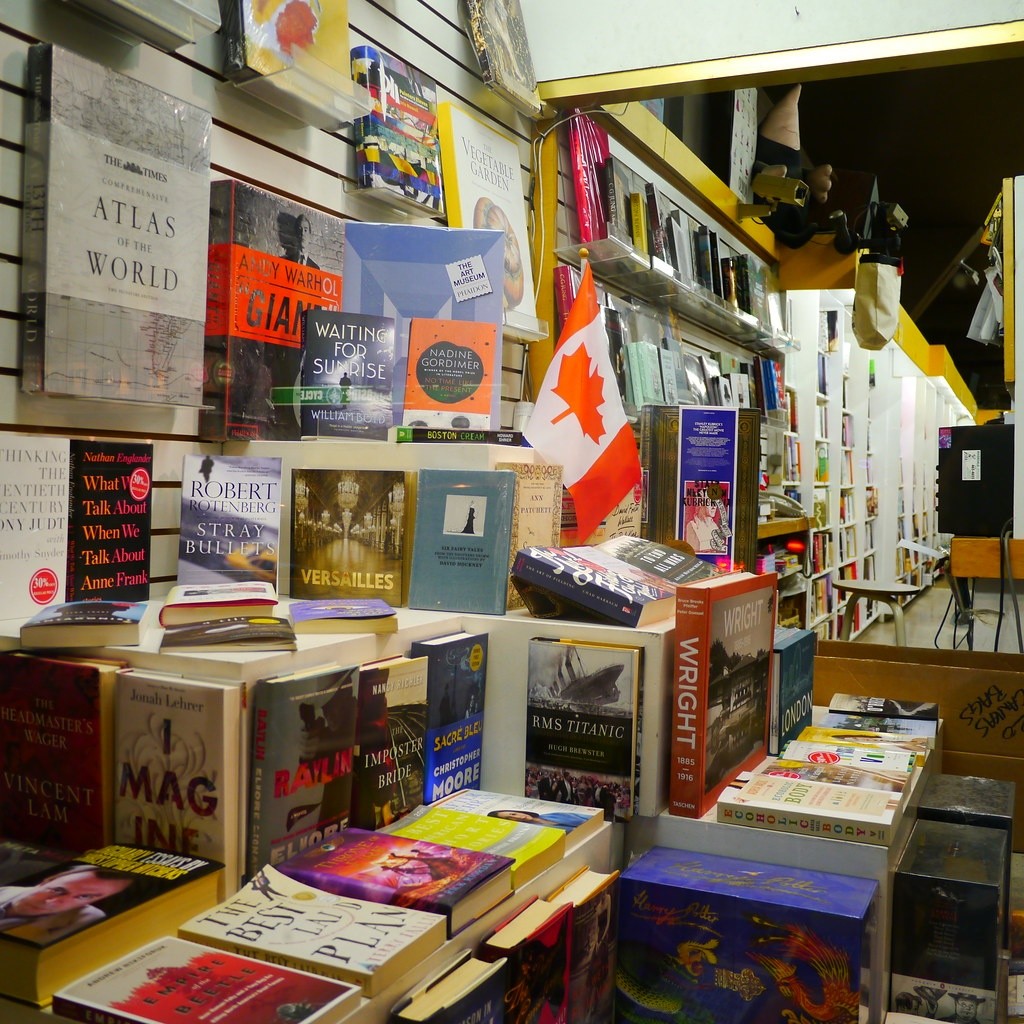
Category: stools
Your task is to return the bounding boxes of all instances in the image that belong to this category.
[831,579,919,647]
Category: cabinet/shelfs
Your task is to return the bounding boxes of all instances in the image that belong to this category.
[0,1,975,1023]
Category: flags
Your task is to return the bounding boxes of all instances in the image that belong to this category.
[524,260,640,538]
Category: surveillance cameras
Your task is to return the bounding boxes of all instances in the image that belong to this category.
[885,203,908,229]
[753,173,809,207]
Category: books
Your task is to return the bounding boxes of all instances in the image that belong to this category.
[82,0,198,53]
[816,442,829,482]
[841,452,853,487]
[672,569,940,846]
[785,436,802,479]
[224,0,354,130]
[553,268,786,415]
[819,358,829,396]
[815,484,877,639]
[349,45,445,217]
[569,112,782,332]
[437,98,536,343]
[464,0,540,117]
[843,413,854,445]
[1,436,563,647]
[1,634,643,1022]
[820,309,839,352]
[513,466,725,628]
[816,405,826,439]
[22,41,503,444]
[786,387,798,432]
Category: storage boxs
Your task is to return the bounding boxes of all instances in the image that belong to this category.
[891,816,1010,1024]
[814,640,1024,855]
[611,846,881,1024]
[917,773,1016,949]
